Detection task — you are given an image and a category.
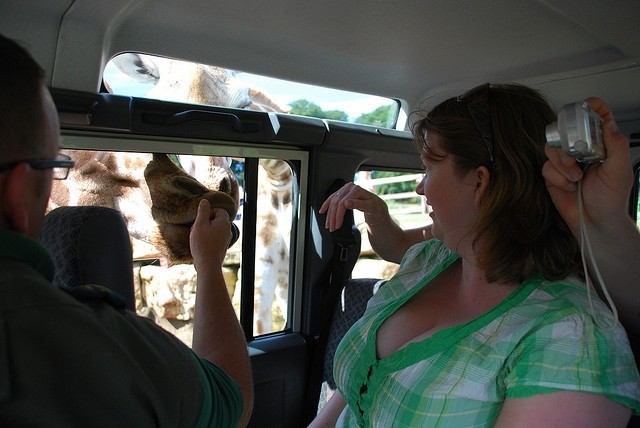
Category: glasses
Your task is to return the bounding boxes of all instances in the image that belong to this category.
[0,153,75,180]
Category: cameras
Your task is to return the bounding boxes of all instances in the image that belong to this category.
[545,101,607,165]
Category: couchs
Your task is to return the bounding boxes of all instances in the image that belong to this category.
[316,278,387,422]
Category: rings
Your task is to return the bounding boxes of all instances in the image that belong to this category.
[336,191,339,196]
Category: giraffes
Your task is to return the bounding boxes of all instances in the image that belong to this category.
[111,53,293,337]
[45,80,240,264]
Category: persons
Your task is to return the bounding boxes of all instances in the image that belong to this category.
[542,92,639,380]
[0,33,256,427]
[317,181,436,266]
[304,83,639,428]
